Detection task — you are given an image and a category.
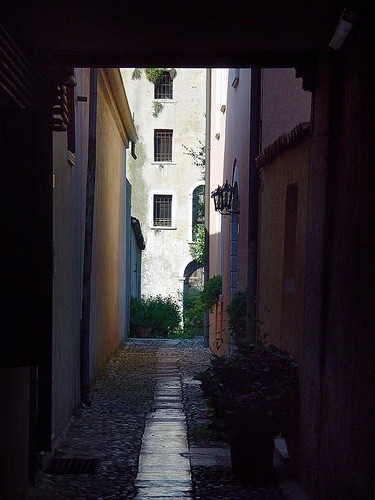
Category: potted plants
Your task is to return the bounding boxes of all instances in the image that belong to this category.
[129,297,181,338]
[152,102,164,117]
[132,70,141,79]
[200,275,223,312]
[146,68,164,85]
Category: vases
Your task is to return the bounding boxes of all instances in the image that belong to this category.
[230,433,275,483]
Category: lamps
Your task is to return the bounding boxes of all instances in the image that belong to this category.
[219,179,240,215]
[211,184,232,215]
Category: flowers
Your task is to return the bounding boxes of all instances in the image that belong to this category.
[192,342,301,444]
